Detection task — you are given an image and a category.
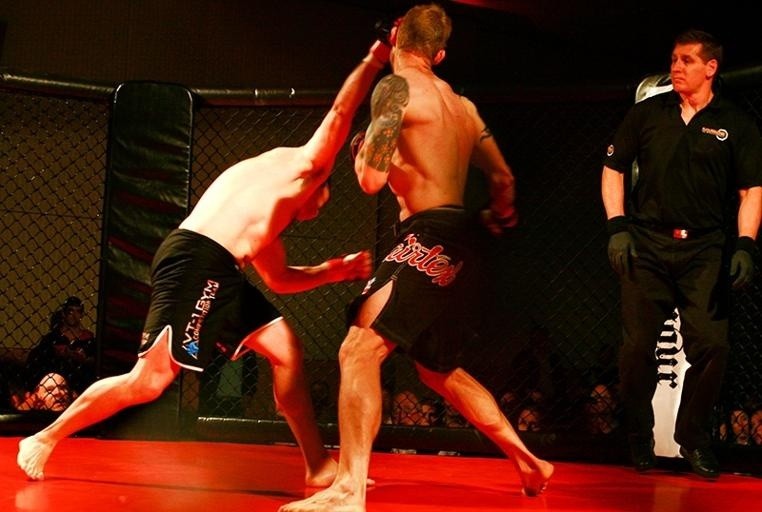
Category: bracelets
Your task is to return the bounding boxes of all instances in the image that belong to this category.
[365,54,385,76]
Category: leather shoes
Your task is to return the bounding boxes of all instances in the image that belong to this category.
[631,435,656,471]
[679,444,721,478]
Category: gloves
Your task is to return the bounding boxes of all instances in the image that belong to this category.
[363,18,393,70]
[729,237,756,290]
[349,129,367,160]
[606,216,639,276]
[328,249,372,283]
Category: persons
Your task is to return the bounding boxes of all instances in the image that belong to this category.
[16,40,390,488]
[22,371,72,412]
[42,296,96,397]
[303,379,759,446]
[196,346,259,418]
[599,28,762,483]
[279,5,558,512]
